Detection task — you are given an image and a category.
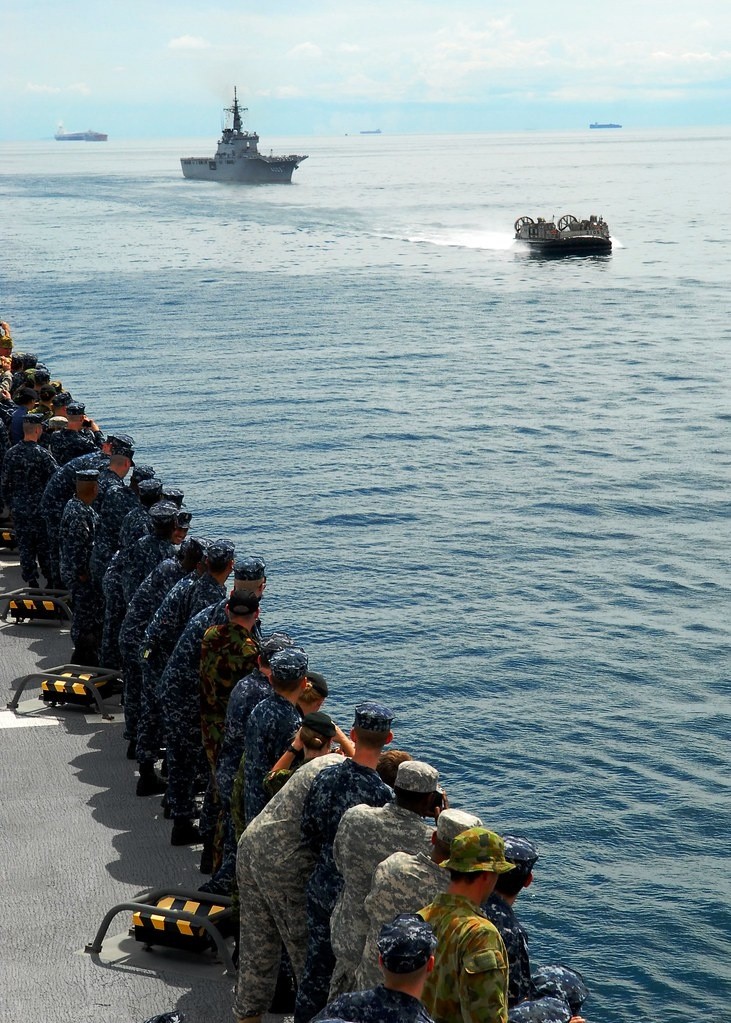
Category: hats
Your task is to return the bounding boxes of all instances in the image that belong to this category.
[507,995,571,1023]
[0,326,539,878]
[528,964,587,1014]
[378,912,439,973]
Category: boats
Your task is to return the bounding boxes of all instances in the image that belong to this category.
[361,128,381,134]
[589,122,623,129]
[180,87,309,183]
[54,122,108,142]
[515,213,612,255]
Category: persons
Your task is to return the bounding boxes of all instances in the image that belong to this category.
[57,465,269,846]
[199,633,592,1023]
[0,320,135,610]
